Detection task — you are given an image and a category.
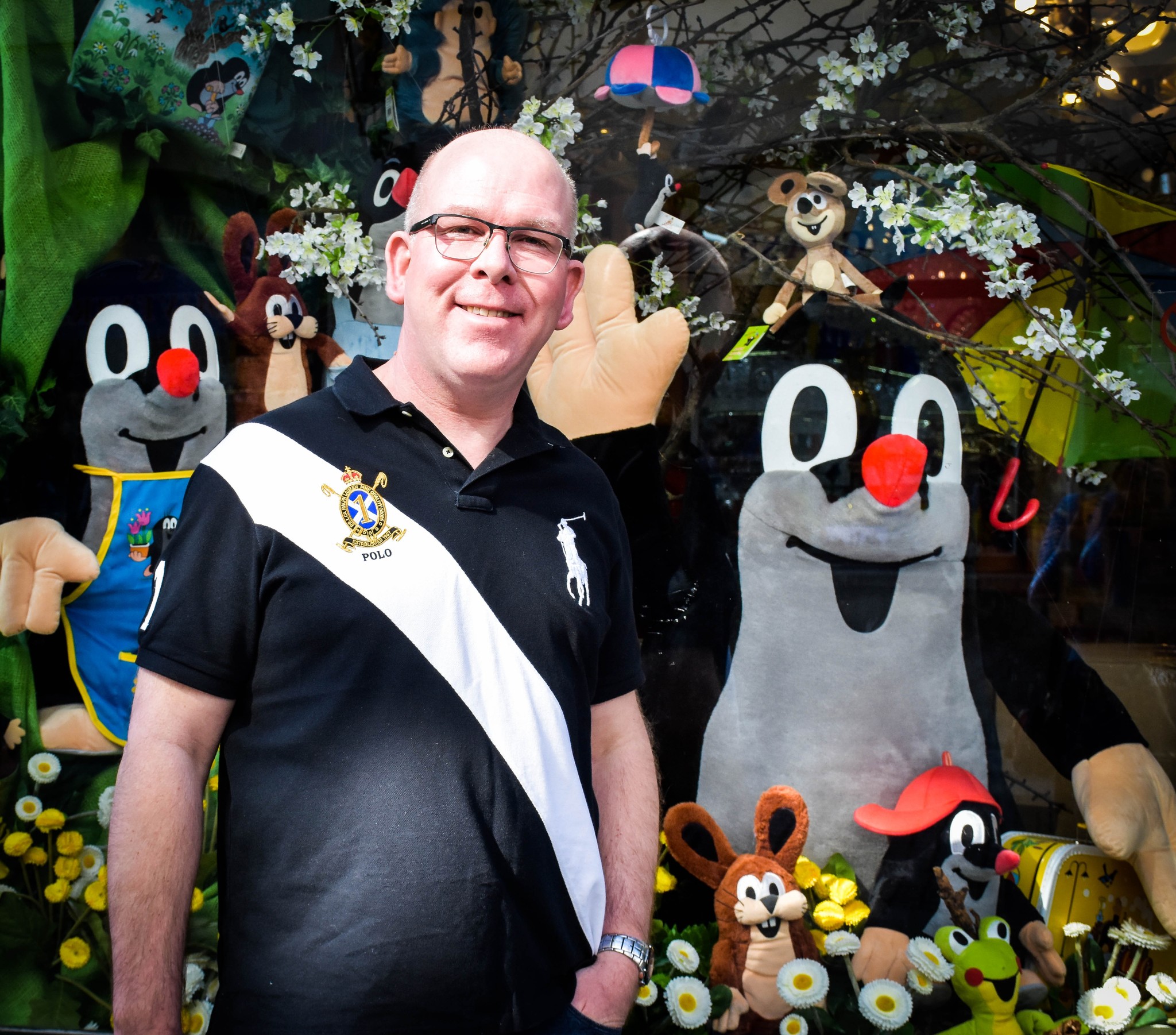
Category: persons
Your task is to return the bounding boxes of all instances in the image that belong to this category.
[106,127,660,1035]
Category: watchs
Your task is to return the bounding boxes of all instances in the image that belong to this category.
[597,932,655,988]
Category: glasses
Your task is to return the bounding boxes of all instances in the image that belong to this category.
[411,213,574,274]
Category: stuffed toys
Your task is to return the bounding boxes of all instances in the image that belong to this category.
[0,263,223,749]
[594,45,711,232]
[381,0,522,141]
[519,244,1176,941]
[225,145,432,443]
[663,751,1066,1035]
[763,172,907,325]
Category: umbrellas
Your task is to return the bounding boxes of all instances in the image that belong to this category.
[846,163,1176,531]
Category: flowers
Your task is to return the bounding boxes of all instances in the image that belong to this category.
[0,749,219,1034]
[633,828,1176,1035]
[234,0,1176,487]
